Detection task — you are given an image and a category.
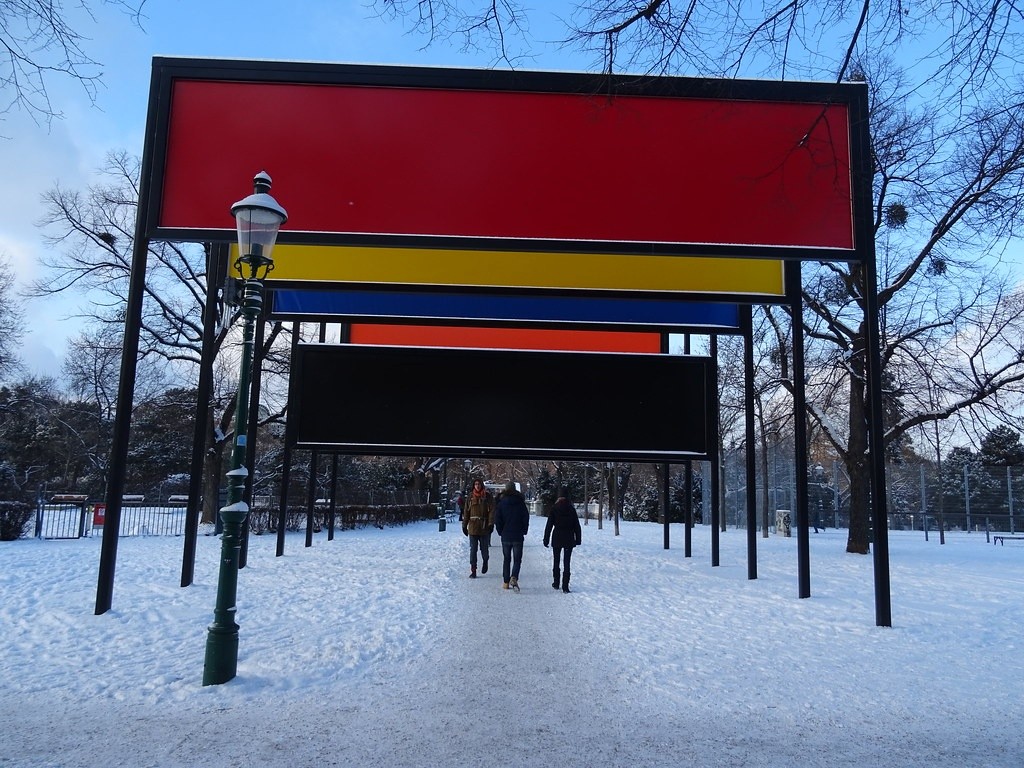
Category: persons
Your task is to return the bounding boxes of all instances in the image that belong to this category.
[738,507,744,528]
[543,487,581,593]
[783,513,791,530]
[462,479,530,594]
[459,491,465,521]
[812,506,826,533]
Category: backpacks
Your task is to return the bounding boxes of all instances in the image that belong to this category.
[458,496,463,507]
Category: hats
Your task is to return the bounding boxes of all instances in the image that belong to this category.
[462,490,465,493]
[557,489,568,498]
[505,482,515,489]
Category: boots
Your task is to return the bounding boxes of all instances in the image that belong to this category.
[562,572,571,592]
[552,568,560,589]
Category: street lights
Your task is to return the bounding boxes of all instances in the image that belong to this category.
[459,459,472,520]
[200,169,289,690]
[768,428,779,534]
[814,462,824,529]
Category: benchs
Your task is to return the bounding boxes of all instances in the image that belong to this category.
[994,536,1024,546]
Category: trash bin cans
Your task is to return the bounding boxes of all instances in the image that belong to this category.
[775,509,791,537]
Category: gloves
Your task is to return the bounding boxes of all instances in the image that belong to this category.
[488,525,493,534]
[463,527,469,537]
[543,539,549,547]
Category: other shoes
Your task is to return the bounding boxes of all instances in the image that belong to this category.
[470,572,476,578]
[511,578,520,591]
[503,582,510,588]
[482,562,488,574]
[459,517,463,521]
